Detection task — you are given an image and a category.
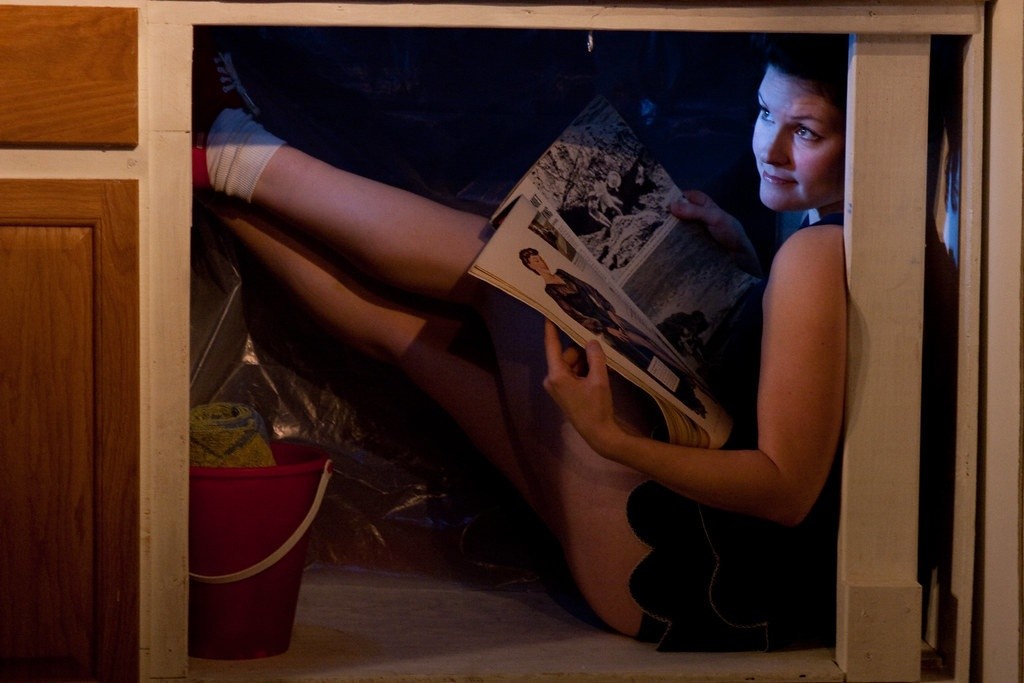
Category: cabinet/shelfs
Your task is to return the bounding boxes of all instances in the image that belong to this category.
[0,2,1024,683]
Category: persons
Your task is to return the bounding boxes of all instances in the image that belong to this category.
[193,30,847,653]
[518,248,710,390]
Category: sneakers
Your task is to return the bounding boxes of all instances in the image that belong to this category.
[191,35,253,187]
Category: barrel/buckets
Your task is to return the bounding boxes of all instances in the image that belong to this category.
[188,442,335,661]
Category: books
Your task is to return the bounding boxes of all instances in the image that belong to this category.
[466,88,757,450]
